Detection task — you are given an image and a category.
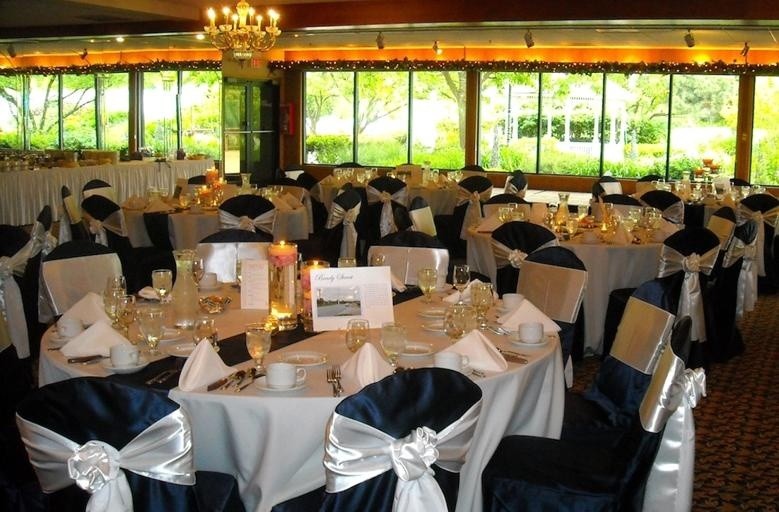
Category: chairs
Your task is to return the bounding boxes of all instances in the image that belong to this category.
[1,140,777,511]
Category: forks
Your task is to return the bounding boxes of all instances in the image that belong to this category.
[325,364,344,398]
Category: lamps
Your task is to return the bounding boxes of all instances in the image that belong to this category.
[738,41,750,57]
[1,37,90,60]
[430,39,442,54]
[370,24,699,50]
[197,1,283,68]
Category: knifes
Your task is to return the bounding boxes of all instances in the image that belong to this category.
[203,369,262,394]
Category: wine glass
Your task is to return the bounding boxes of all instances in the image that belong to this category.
[497,170,766,247]
[103,269,219,357]
[417,263,496,346]
[330,165,464,190]
[243,321,273,375]
[346,321,407,372]
[129,173,294,211]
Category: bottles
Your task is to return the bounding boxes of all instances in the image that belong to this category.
[169,248,201,320]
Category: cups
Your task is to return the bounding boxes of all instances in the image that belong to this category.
[269,240,329,336]
[267,363,306,388]
[337,255,387,267]
[109,346,139,368]
[435,352,468,373]
[500,292,546,342]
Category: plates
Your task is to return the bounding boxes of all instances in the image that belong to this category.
[278,351,330,368]
[382,339,436,359]
[252,376,308,391]
[508,338,548,346]
[425,363,473,379]
[102,361,148,371]
